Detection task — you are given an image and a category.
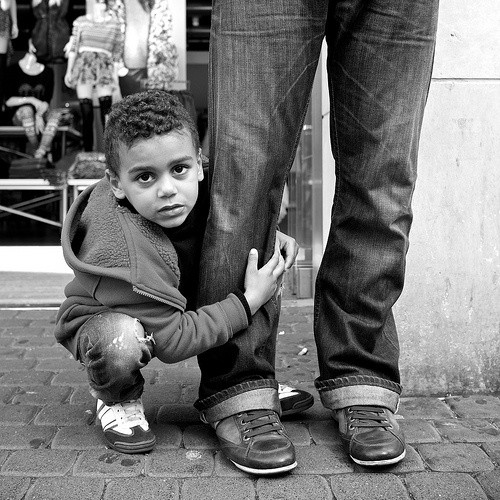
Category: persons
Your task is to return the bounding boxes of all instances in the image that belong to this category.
[54,91,313,455]
[194,1,437,477]
[0,0,127,179]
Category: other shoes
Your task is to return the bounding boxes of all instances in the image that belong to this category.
[213,409,301,471]
[277,384,317,416]
[94,398,158,455]
[336,405,411,465]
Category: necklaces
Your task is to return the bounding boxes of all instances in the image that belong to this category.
[89,12,107,29]
